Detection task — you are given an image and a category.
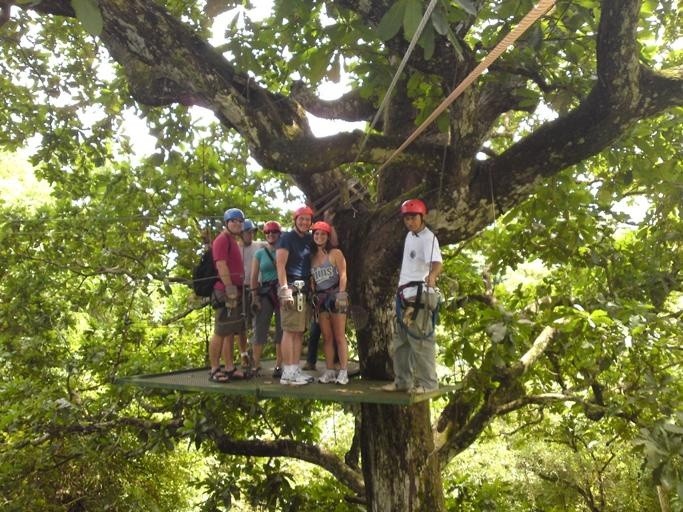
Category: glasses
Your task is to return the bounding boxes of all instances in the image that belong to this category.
[265,230,279,234]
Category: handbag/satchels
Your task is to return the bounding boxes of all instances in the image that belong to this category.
[263,247,288,311]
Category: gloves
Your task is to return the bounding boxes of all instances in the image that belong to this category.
[335,292,349,314]
[277,286,294,312]
[224,285,239,308]
[250,297,261,317]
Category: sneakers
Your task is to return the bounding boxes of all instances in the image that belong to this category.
[279,365,314,385]
[335,369,349,385]
[382,380,414,392]
[406,385,435,394]
[318,369,336,384]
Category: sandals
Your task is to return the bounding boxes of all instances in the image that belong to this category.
[208,368,229,383]
[224,367,248,380]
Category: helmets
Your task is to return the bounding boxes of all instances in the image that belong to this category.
[263,221,282,245]
[223,208,244,236]
[293,206,313,234]
[311,221,331,247]
[240,218,258,247]
[401,199,426,233]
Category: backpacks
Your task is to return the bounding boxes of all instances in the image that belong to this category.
[192,232,232,297]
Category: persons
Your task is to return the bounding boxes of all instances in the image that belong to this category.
[249,220,284,378]
[236,218,269,368]
[275,207,318,386]
[309,220,351,386]
[381,198,444,395]
[206,207,253,383]
